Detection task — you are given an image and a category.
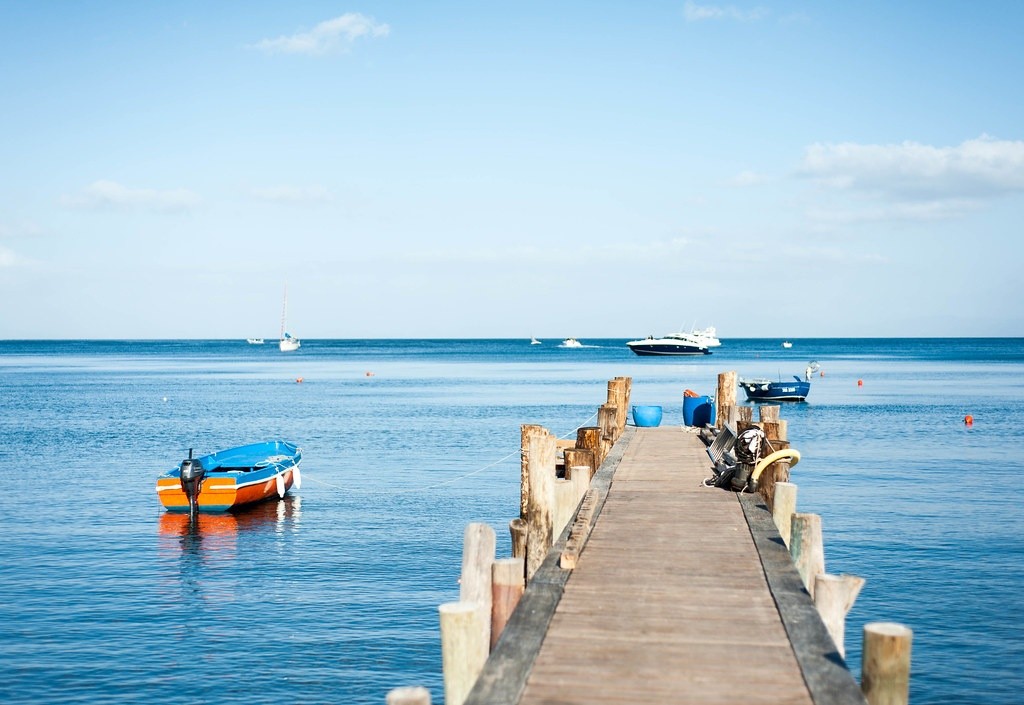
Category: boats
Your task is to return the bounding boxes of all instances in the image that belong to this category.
[156,439,303,515]
[784,343,791,347]
[562,339,580,348]
[626,325,722,358]
[740,367,812,402]
[531,338,542,346]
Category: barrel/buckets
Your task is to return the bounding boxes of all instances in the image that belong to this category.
[683,396,716,428]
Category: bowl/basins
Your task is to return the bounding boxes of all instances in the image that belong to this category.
[633,406,662,427]
